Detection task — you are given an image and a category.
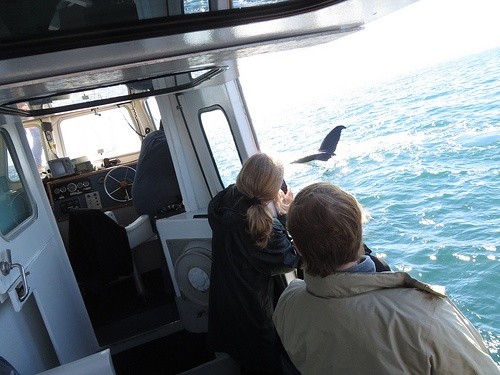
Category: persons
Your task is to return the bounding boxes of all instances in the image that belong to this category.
[205,152,302,375]
[272,183,500,375]
[132,117,192,264]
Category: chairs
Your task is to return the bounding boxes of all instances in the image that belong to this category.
[71,204,162,301]
[129,131,185,217]
